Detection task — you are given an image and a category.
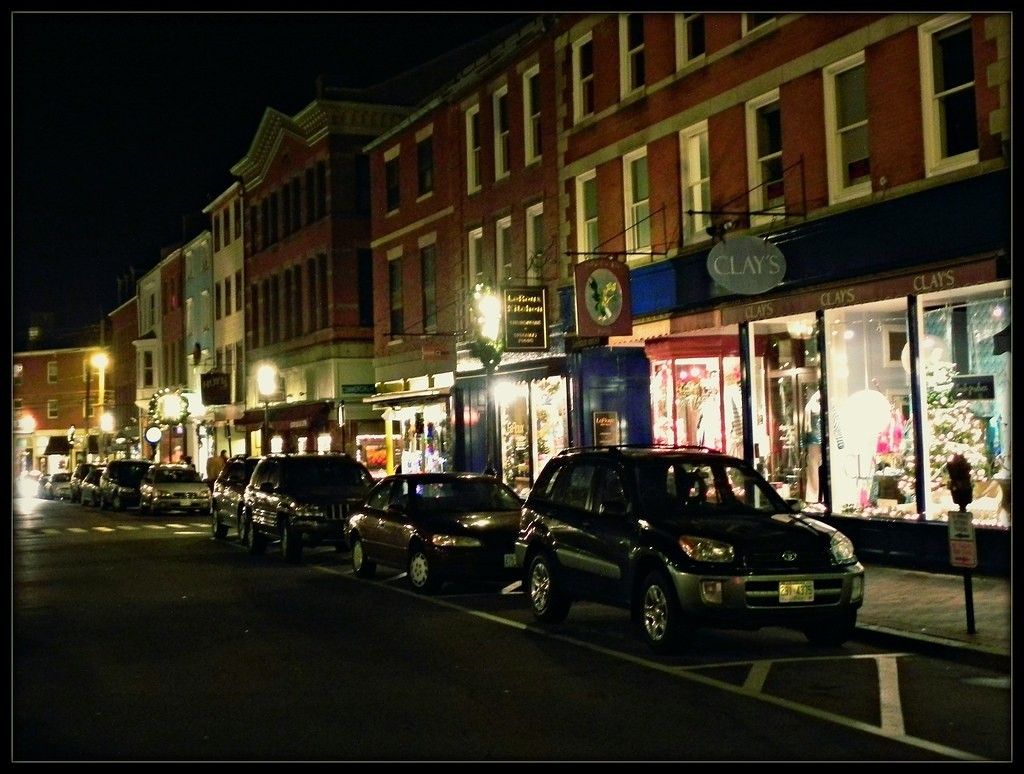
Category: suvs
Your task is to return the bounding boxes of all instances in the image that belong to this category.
[514,444,863,654]
[211,455,267,539]
[238,455,375,561]
[99,457,148,510]
[141,463,211,515]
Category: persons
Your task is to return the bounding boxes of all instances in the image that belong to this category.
[803,390,820,502]
[219,450,229,462]
[185,456,195,468]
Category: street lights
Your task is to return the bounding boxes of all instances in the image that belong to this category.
[477,290,500,476]
[259,366,272,455]
[163,396,177,463]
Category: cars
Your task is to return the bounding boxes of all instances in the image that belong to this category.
[344,473,523,592]
[72,463,104,505]
[34,472,72,500]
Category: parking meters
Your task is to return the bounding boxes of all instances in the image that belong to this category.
[945,454,977,633]
